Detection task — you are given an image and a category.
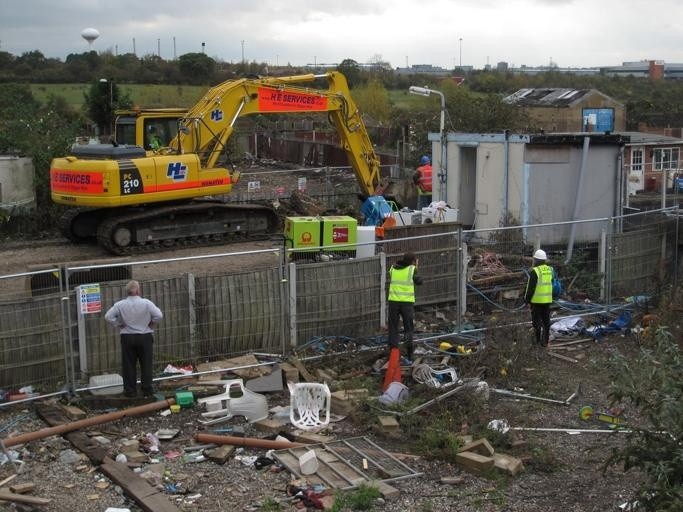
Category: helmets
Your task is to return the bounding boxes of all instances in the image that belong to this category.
[532,248,548,260]
[421,155,430,165]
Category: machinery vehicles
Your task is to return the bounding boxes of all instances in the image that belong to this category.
[45,66,389,263]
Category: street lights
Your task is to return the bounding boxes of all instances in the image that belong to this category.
[115,45,118,56]
[98,75,114,137]
[460,38,463,70]
[406,83,447,205]
[406,56,408,67]
[157,38,161,56]
[314,56,317,65]
[173,36,176,59]
[133,37,135,54]
[277,54,279,65]
[241,39,245,64]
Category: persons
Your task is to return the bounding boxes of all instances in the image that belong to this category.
[413,155,432,209]
[103,280,162,398]
[524,249,554,347]
[386,250,424,355]
[145,128,162,149]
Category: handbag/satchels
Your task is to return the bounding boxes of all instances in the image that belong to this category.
[552,278,561,297]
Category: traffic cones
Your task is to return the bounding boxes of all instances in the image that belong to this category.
[376,347,404,395]
